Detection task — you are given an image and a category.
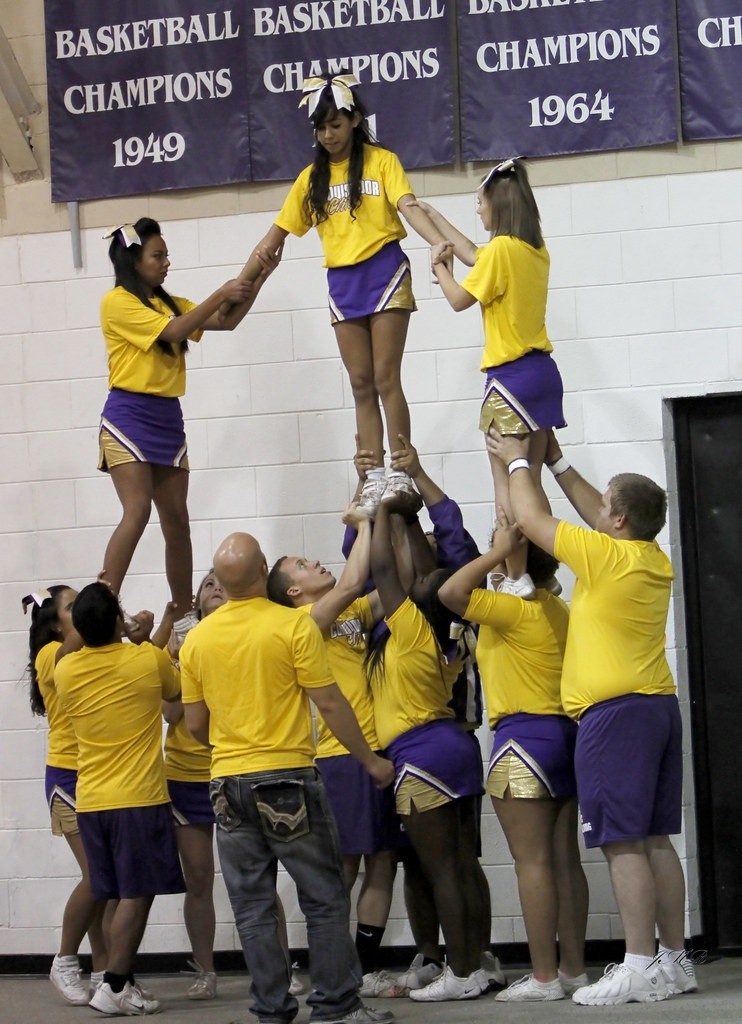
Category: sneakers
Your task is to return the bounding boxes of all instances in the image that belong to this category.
[481,958,507,990]
[396,954,443,991]
[180,958,216,1000]
[495,973,565,1001]
[117,595,139,636]
[88,981,161,1016]
[492,572,535,599]
[89,969,154,1000]
[379,471,413,500]
[172,611,200,644]
[544,575,562,597]
[558,969,589,993]
[359,970,402,998]
[308,1003,393,1024]
[288,962,304,995]
[657,959,697,994]
[357,477,387,517]
[572,962,671,1006]
[49,953,89,1005]
[410,955,481,1002]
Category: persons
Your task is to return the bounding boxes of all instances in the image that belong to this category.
[180,534,395,1024]
[151,568,305,999]
[341,434,502,1001]
[438,420,701,1006]
[96,218,285,623]
[406,156,568,599]
[266,501,402,1000]
[20,585,107,1008]
[198,74,456,518]
[53,583,182,1017]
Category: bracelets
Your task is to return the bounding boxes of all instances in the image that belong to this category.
[548,456,572,476]
[508,458,531,476]
